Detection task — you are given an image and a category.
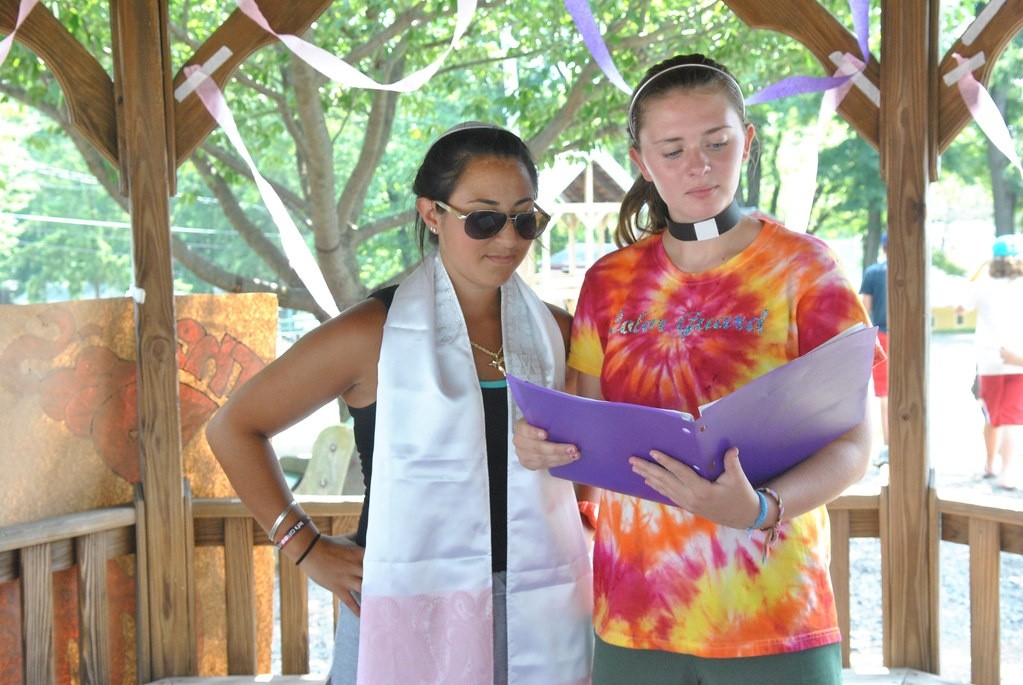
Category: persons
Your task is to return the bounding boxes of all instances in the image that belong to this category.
[206,121,604,685]
[512,54,871,685]
[975,234,1023,490]
[859,230,889,469]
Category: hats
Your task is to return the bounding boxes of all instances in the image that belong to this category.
[882,232,888,247]
[993,235,1023,256]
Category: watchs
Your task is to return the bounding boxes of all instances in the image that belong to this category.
[577,501,599,526]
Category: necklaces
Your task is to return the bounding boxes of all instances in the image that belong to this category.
[470,341,507,377]
[665,200,741,242]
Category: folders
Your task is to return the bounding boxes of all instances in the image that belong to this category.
[506,323,881,508]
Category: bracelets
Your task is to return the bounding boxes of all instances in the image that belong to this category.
[276,516,311,549]
[756,487,784,566]
[295,534,320,565]
[269,500,298,542]
[748,490,767,531]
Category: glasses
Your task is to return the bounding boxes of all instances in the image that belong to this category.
[435,202,550,241]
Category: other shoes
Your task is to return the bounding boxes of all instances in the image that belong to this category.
[873,447,889,466]
[983,471,1000,479]
[996,482,1018,491]
